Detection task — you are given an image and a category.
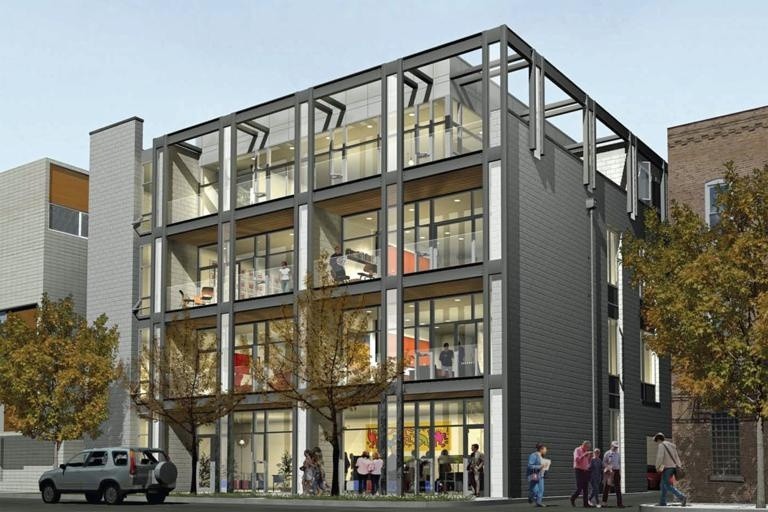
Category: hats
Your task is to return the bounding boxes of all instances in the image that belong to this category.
[610,441,619,448]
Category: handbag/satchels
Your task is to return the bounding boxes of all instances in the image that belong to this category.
[674,467,686,481]
[530,473,539,482]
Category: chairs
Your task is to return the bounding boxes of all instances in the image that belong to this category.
[271,475,285,492]
[179,290,193,309]
[199,286,213,305]
[357,263,377,280]
[330,268,349,284]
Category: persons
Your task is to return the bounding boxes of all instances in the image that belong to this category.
[277,261,292,293]
[653,432,688,506]
[439,341,455,377]
[329,245,347,279]
[300,440,483,498]
[527,442,551,507]
[570,440,593,509]
[459,341,465,362]
[588,447,602,508]
[603,440,627,507]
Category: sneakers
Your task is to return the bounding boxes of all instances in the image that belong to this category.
[584,501,608,508]
[537,503,545,507]
[681,495,687,506]
[528,496,532,503]
[655,503,667,506]
[617,503,624,507]
[570,499,576,507]
